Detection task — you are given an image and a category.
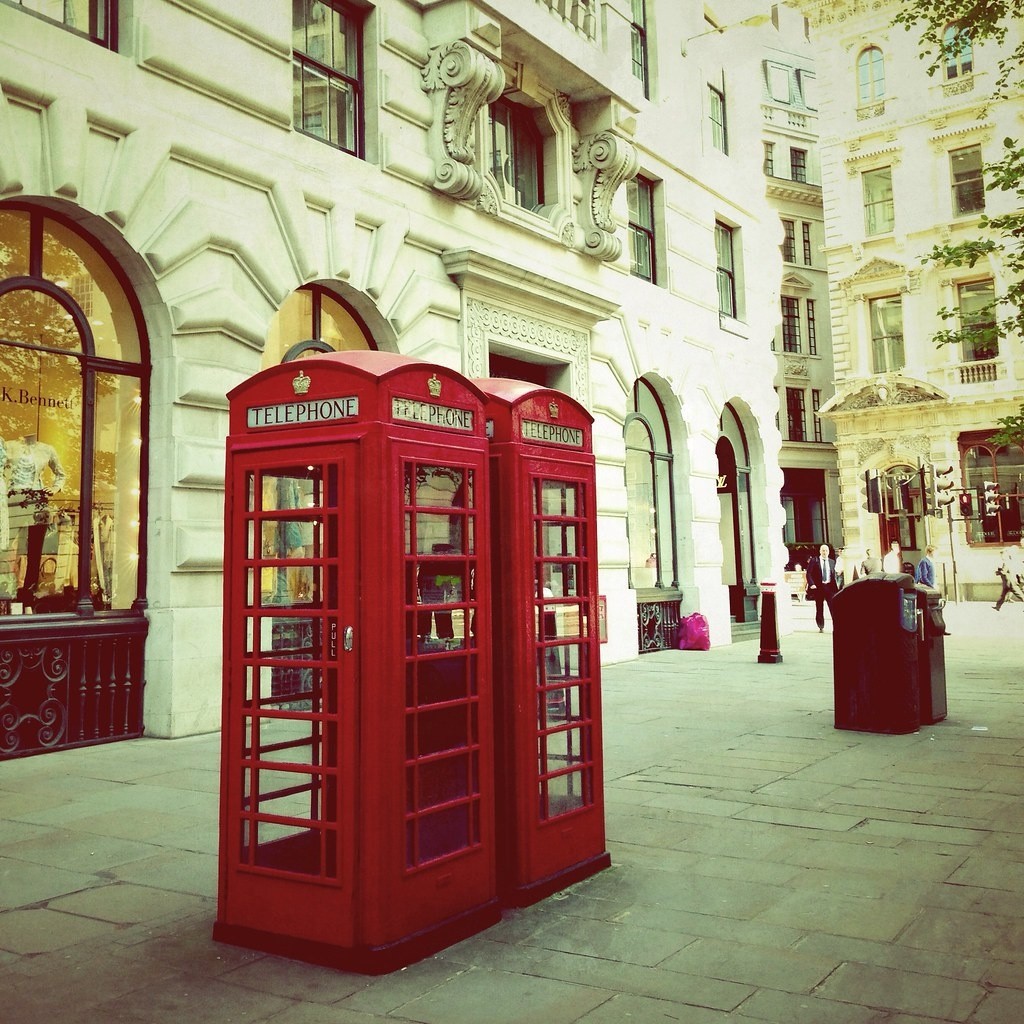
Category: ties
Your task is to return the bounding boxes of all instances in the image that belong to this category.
[822,560,827,582]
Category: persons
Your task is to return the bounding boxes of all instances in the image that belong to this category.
[1004,545,1024,603]
[834,548,844,590]
[992,549,1024,612]
[273,476,303,607]
[916,544,952,636]
[883,540,903,574]
[0,435,11,552]
[1020,538,1024,547]
[806,545,840,633]
[410,549,555,643]
[860,549,882,575]
[5,433,66,613]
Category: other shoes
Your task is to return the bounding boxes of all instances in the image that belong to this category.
[944,632,951,636]
[992,606,999,611]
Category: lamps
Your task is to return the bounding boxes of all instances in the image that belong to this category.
[680,15,772,57]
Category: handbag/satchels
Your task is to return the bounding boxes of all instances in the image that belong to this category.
[678,613,710,650]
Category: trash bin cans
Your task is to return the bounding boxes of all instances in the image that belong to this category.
[831,573,947,734]
